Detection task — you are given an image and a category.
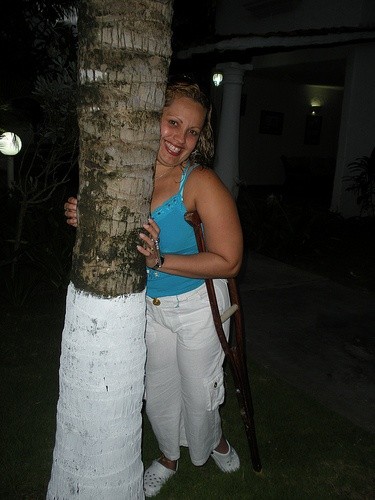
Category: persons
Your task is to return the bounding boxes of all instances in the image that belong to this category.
[65,82,242,496]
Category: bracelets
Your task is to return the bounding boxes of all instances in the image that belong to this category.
[147,256,164,270]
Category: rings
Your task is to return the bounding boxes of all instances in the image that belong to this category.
[147,223,162,268]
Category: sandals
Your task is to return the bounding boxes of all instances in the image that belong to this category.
[210,438,240,473]
[141,457,179,498]
